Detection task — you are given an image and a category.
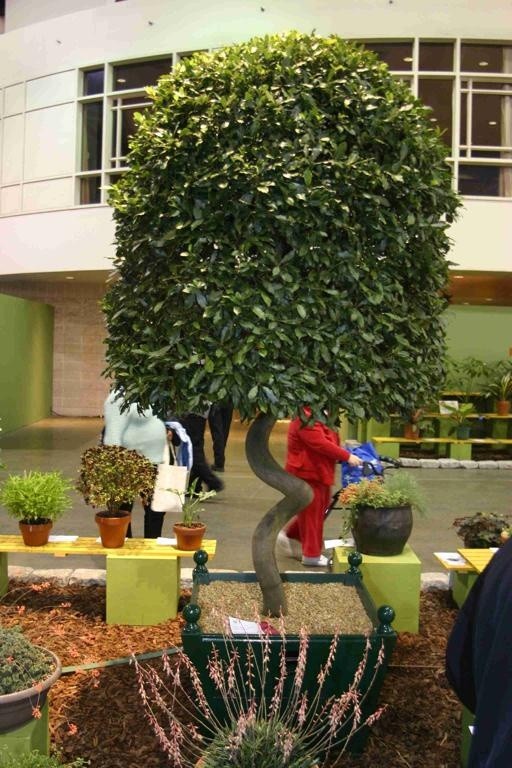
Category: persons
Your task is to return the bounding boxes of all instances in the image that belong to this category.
[283,404,363,566]
[212,392,233,471]
[104,378,182,541]
[169,394,222,498]
[444,537,511,768]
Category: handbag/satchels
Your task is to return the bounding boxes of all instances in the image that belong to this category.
[150,436,191,514]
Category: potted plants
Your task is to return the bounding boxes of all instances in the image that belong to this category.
[93,27,470,748]
[74,443,158,549]
[458,355,512,415]
[0,630,62,734]
[119,605,395,768]
[160,477,218,551]
[441,355,486,415]
[393,407,429,439]
[0,466,77,546]
[440,402,477,439]
[336,471,430,557]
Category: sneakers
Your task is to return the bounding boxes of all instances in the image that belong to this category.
[302,553,333,566]
[277,530,293,558]
